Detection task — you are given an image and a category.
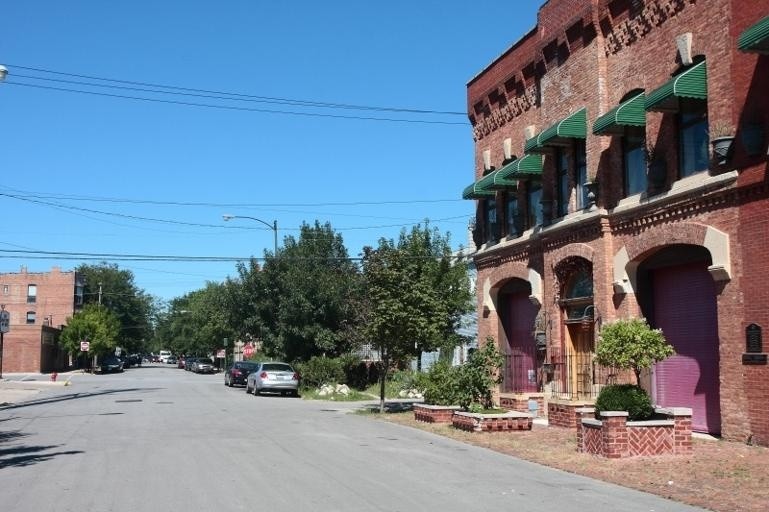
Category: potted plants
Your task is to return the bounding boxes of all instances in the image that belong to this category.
[539,191,553,217]
[639,124,673,188]
[582,174,601,205]
[703,118,738,167]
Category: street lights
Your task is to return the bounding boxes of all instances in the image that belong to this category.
[222,212,279,263]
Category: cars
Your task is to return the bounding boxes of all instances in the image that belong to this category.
[245,360,299,396]
[102,350,215,373]
[224,361,258,387]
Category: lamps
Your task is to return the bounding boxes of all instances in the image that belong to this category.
[579,305,602,334]
